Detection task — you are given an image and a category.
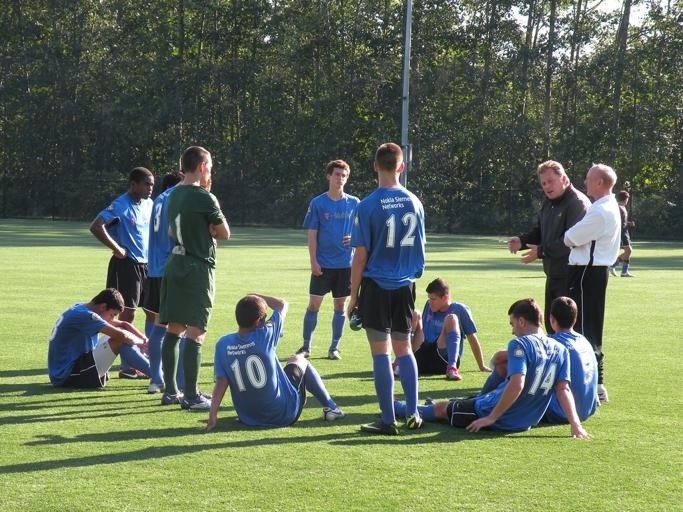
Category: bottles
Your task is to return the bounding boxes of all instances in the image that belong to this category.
[349,305,363,333]
[425,397,434,405]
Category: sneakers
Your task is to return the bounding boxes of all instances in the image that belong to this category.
[119,367,223,410]
[620,272,637,277]
[593,383,609,408]
[392,361,400,378]
[361,416,399,435]
[322,406,346,422]
[445,365,461,380]
[608,267,618,279]
[328,347,341,359]
[406,413,425,431]
[295,346,312,358]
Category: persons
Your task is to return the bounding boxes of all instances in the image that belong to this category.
[162,171,182,187]
[467,297,598,423]
[47,288,163,388]
[89,167,156,378]
[141,179,214,399]
[505,160,592,332]
[158,145,231,408]
[609,190,636,277]
[392,277,493,379]
[202,293,344,433]
[563,163,622,403]
[290,158,360,358]
[345,143,422,434]
[393,298,592,439]
